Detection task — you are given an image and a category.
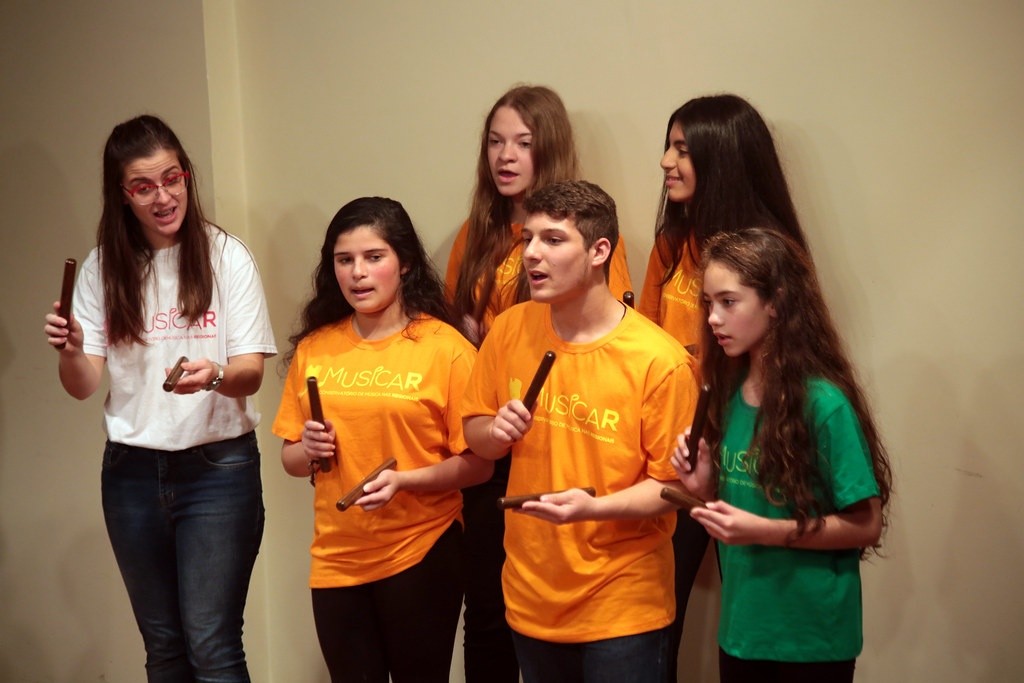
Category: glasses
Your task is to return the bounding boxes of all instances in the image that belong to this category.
[119,169,190,205]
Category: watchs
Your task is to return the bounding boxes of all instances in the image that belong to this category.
[201,361,224,392]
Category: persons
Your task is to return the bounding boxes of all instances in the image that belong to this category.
[45,115,278,683]
[444,86,894,683]
[272,197,494,683]
